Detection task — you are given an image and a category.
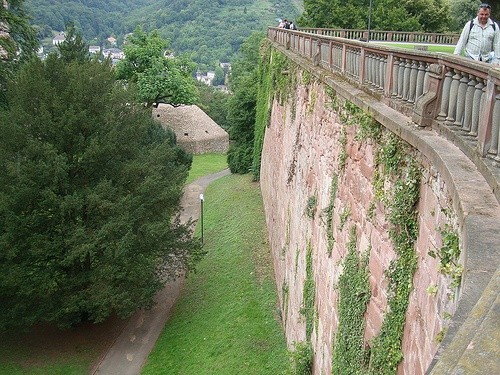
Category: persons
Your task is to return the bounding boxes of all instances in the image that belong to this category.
[454,4,500,66]
[283,18,291,29]
[289,21,297,30]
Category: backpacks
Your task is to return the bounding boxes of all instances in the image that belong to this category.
[286,23,290,29]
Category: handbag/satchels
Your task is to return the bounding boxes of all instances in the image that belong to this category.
[294,25,296,30]
